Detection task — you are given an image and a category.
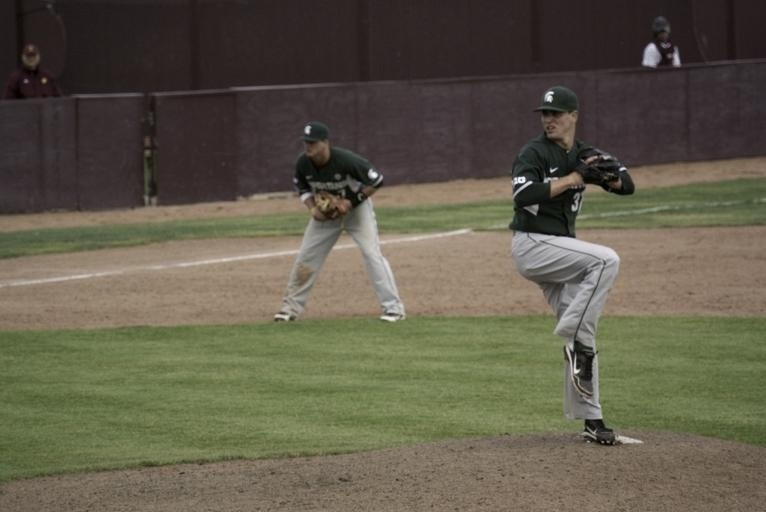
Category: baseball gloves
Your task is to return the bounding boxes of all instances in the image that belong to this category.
[573,146,621,183]
[313,190,346,223]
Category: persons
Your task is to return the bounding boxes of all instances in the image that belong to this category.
[273,120,408,323]
[641,15,682,70]
[506,84,637,446]
[1,42,68,99]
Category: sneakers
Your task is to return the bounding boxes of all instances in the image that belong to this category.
[379,311,406,322]
[274,312,296,321]
[582,422,615,445]
[563,339,596,399]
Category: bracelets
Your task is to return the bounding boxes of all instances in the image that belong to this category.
[345,190,369,208]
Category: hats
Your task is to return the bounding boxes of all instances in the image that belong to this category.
[300,121,330,142]
[532,86,578,113]
